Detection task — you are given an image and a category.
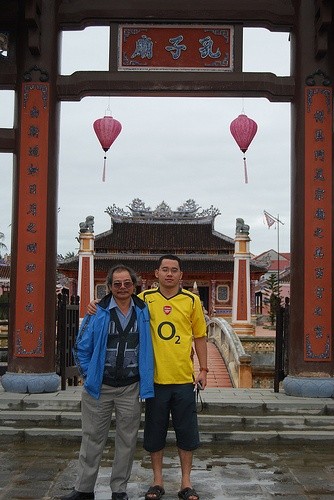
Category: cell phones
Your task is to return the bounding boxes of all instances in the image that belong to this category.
[196,381,204,390]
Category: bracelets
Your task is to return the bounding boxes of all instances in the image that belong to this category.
[199,368,209,373]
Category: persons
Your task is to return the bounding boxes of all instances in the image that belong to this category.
[51,266,154,500]
[87,255,209,500]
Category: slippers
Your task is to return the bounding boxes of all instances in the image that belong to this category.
[145,484,165,500]
[178,487,200,500]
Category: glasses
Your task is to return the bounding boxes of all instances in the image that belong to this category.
[111,281,134,290]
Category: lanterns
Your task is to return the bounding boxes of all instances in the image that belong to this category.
[231,114,257,185]
[94,116,122,183]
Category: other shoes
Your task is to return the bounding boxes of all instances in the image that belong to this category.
[60,489,95,500]
[112,492,128,500]
